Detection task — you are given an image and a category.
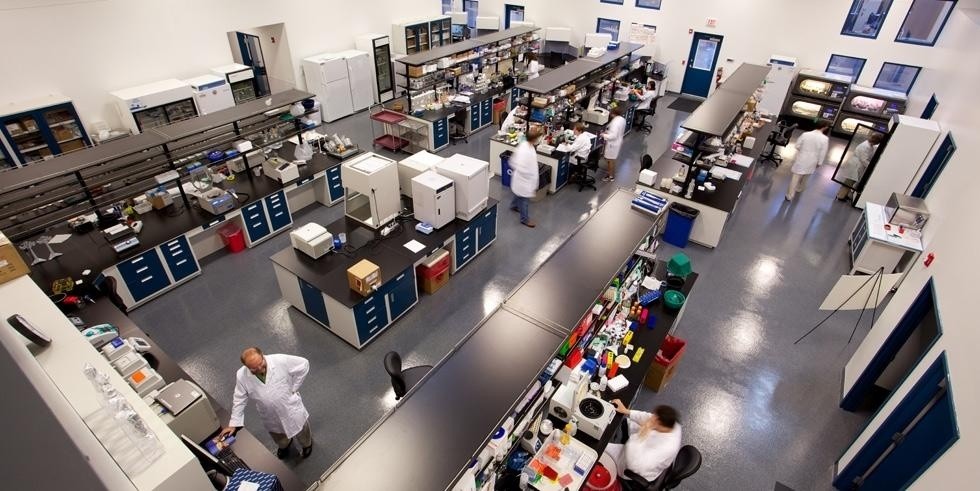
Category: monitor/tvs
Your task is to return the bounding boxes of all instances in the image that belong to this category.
[179,432,234,478]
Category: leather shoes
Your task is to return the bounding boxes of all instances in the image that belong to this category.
[835,194,852,203]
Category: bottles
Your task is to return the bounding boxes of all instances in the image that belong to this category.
[598,362,609,392]
[698,170,714,182]
[552,415,579,448]
[677,164,686,176]
[333,233,342,249]
[501,87,587,144]
[519,472,529,491]
[628,288,662,324]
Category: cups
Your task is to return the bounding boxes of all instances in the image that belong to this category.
[338,233,347,243]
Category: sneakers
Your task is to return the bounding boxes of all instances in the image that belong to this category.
[301,439,313,459]
[784,194,792,203]
[601,174,615,182]
[509,206,537,229]
[276,437,293,460]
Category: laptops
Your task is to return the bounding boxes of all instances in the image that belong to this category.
[154,378,202,417]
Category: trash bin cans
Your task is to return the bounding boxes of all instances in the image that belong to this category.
[499,150,552,201]
[216,223,245,253]
[643,333,687,393]
[493,98,506,125]
[663,202,700,248]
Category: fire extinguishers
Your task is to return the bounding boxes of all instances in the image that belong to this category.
[715,67,723,83]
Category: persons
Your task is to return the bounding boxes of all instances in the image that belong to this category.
[510,123,542,229]
[782,121,829,204]
[567,124,593,169]
[499,105,531,132]
[522,51,542,78]
[632,78,656,115]
[218,346,316,458]
[603,396,681,484]
[834,130,881,199]
[596,107,628,183]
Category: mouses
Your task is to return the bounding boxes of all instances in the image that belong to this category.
[218,434,229,443]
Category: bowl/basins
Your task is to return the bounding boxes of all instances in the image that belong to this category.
[206,149,226,162]
[302,99,315,108]
[664,290,686,308]
